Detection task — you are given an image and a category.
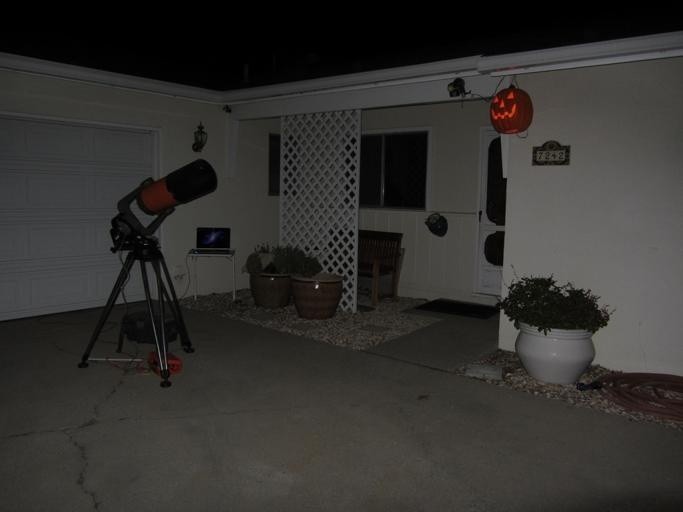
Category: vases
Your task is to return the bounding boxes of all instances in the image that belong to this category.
[293,274,343,319]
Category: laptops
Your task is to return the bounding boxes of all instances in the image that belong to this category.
[194,226,231,254]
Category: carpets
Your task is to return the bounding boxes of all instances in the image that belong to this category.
[416,298,496,320]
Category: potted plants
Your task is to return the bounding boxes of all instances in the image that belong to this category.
[243,241,306,308]
[501,271,618,387]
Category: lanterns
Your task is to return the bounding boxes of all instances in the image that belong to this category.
[486,82,532,137]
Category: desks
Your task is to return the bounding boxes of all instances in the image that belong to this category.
[188,253,236,301]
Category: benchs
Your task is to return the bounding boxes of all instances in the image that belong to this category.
[358,230,403,306]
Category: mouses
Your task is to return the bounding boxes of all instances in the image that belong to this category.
[194,250,199,253]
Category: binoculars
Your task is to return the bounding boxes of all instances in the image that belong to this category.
[105,158,218,252]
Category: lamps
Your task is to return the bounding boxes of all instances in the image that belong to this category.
[193,120,207,152]
[448,78,471,97]
[489,85,533,134]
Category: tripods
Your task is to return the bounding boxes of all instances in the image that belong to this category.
[77,240,195,387]
